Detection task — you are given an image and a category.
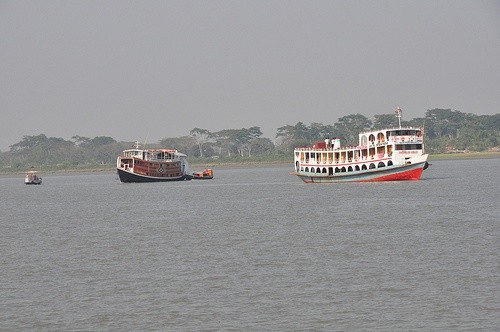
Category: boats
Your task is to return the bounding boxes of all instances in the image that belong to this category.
[116,141,193,183]
[191,168,214,179]
[289,125,432,182]
[24,166,42,185]
